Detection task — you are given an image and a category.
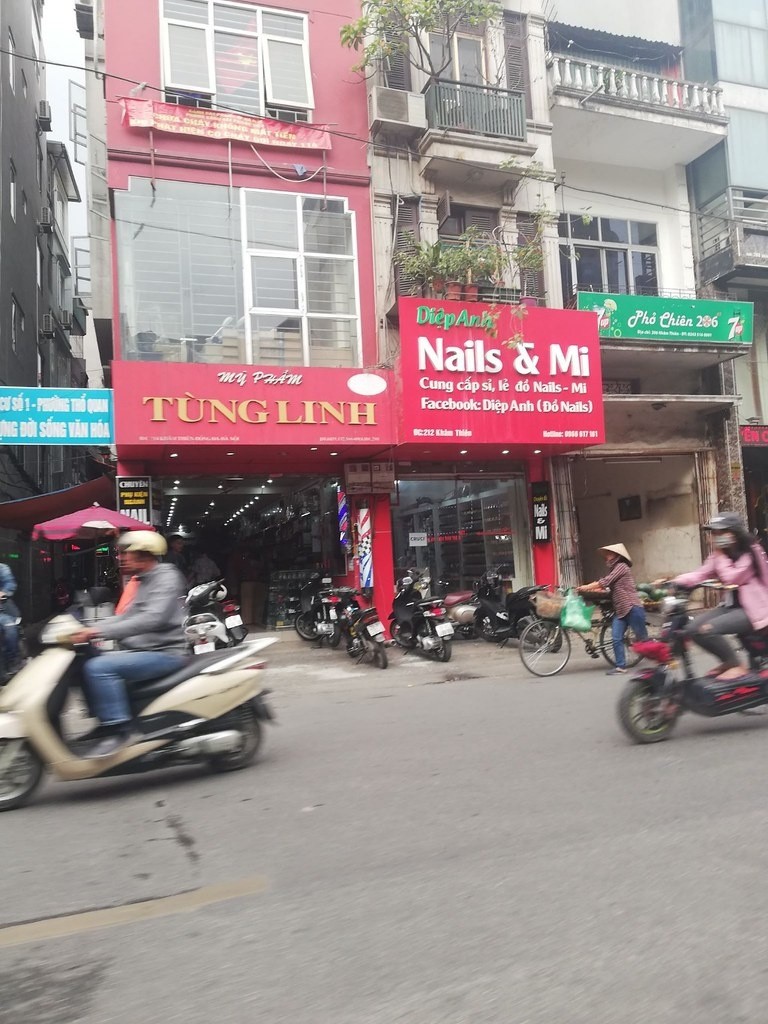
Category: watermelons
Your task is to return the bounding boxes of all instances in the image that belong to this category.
[634,582,670,601]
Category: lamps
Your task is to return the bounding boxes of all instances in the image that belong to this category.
[745,416,761,425]
[356,499,374,598]
[337,485,353,554]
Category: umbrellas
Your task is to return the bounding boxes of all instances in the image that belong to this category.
[32,501,156,588]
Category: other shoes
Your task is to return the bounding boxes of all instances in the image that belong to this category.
[85,733,131,758]
[605,668,625,675]
[715,666,749,680]
[709,663,731,676]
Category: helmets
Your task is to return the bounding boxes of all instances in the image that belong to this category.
[169,523,196,547]
[210,584,227,603]
[119,528,168,556]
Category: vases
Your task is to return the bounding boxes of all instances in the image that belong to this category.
[481,108,512,139]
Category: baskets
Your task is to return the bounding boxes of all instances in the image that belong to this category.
[536,590,566,619]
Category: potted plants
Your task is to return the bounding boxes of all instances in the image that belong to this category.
[339,0,504,134]
[391,199,594,306]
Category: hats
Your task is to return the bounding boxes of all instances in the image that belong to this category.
[700,512,741,530]
[596,542,633,567]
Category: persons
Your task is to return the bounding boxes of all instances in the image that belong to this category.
[576,543,668,674]
[66,529,188,759]
[187,546,221,588]
[649,511,768,680]
[1,562,21,664]
[163,534,190,576]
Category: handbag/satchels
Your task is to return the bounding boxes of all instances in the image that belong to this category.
[560,588,594,633]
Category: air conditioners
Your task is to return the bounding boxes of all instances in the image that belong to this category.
[40,206,54,233]
[367,85,426,139]
[43,314,55,339]
[37,100,52,132]
[61,308,73,329]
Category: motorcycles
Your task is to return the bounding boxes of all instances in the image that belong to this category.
[473,564,563,653]
[177,584,248,649]
[180,577,230,655]
[407,570,482,640]
[320,586,397,669]
[0,602,280,812]
[295,567,341,647]
[388,573,455,662]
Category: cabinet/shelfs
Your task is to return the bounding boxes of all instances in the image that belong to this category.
[238,510,324,574]
[395,486,531,594]
[265,578,303,630]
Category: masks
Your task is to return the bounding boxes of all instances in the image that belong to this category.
[713,534,734,547]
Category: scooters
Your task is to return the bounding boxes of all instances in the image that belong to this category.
[615,579,768,744]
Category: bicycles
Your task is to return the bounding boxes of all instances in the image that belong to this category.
[518,586,645,677]
